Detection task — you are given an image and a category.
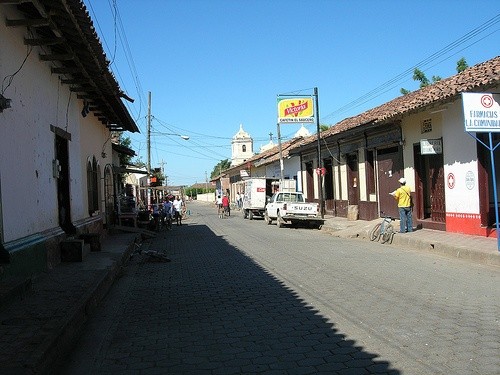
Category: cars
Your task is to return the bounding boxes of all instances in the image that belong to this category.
[136,203,164,232]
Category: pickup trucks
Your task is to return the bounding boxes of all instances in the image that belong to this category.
[263,191,321,228]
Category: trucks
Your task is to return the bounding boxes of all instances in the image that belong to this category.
[237,177,297,221]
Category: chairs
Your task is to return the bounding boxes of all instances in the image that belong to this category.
[79,233,101,251]
[61,237,90,262]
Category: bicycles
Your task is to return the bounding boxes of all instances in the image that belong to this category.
[369,211,395,244]
[129,236,171,262]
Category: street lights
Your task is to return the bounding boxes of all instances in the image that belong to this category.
[147,91,189,211]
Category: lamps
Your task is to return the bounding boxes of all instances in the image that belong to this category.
[81,100,89,118]
[0,94,12,113]
[102,152,107,159]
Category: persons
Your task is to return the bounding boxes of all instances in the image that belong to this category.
[394,177,413,233]
[214,188,245,216]
[151,193,185,231]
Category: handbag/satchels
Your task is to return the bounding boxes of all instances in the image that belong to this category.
[410,196,414,207]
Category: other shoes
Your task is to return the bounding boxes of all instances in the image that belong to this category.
[396,230,402,233]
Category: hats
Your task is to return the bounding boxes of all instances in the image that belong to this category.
[398,178,406,184]
[223,195,226,196]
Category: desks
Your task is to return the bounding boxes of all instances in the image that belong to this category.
[138,221,150,228]
[117,213,137,227]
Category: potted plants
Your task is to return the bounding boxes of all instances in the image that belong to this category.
[138,211,149,221]
